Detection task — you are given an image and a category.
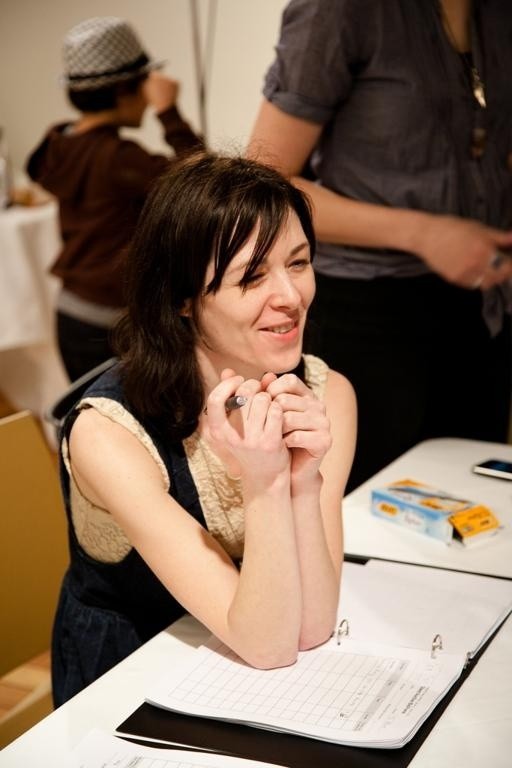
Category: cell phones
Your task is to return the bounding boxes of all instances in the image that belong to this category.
[474,458,512,480]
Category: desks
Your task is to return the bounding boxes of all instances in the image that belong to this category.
[2,432,512,768]
[1,201,72,441]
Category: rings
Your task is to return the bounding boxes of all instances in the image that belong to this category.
[489,250,507,269]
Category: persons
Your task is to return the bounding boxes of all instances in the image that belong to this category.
[50,148,358,707]
[22,14,210,383]
[246,0,512,493]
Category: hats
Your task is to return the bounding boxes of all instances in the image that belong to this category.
[57,14,171,90]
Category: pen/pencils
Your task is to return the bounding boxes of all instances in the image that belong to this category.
[203,396,248,415]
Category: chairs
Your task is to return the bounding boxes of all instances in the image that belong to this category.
[1,406,90,749]
